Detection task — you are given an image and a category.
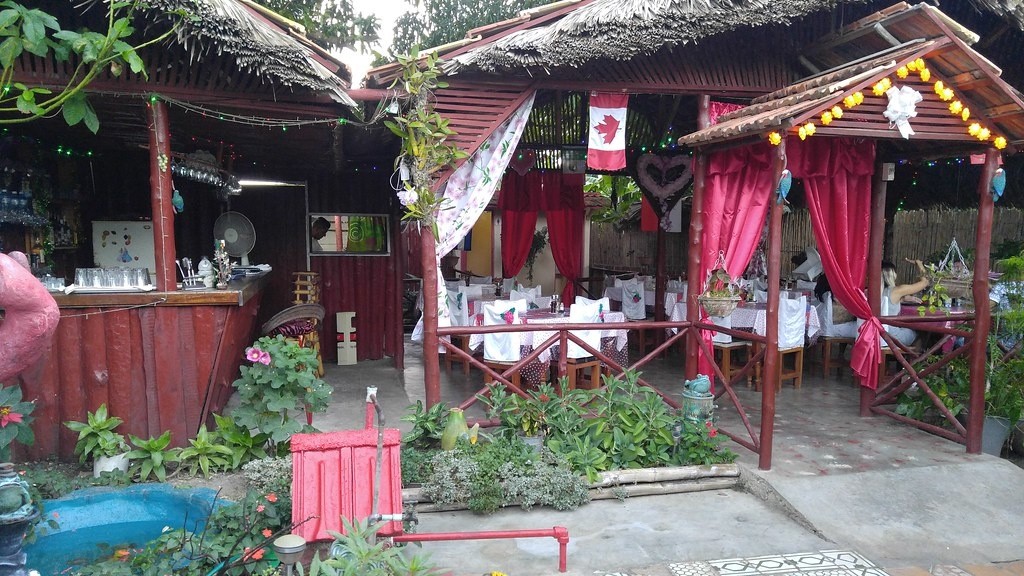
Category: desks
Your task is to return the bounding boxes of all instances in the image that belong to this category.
[468,309,629,387]
[670,297,807,391]
[900,303,970,354]
[603,285,683,348]
[452,291,552,349]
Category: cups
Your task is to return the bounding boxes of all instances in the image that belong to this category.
[34,276,66,291]
[74,267,152,288]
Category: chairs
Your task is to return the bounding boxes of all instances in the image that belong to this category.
[260,303,327,377]
[418,272,917,414]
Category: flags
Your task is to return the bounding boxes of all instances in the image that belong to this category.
[587,94,629,170]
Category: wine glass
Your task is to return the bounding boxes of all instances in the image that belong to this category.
[170,151,224,187]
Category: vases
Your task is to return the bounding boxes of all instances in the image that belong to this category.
[931,277,970,300]
[515,431,544,454]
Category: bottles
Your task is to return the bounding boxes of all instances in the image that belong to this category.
[0,189,39,224]
[555,295,560,312]
[48,209,71,239]
[550,295,557,313]
[32,237,46,269]
[198,256,213,288]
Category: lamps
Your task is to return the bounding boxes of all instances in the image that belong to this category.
[274,534,307,565]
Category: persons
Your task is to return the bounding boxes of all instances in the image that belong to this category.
[815,274,854,324]
[312,217,330,251]
[882,260,929,361]
[987,280,1024,353]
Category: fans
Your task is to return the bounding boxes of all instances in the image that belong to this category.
[214,211,256,265]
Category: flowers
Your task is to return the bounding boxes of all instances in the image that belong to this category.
[916,262,1006,316]
[476,376,559,435]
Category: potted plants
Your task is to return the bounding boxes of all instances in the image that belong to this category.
[62,403,131,479]
[894,330,1024,457]
